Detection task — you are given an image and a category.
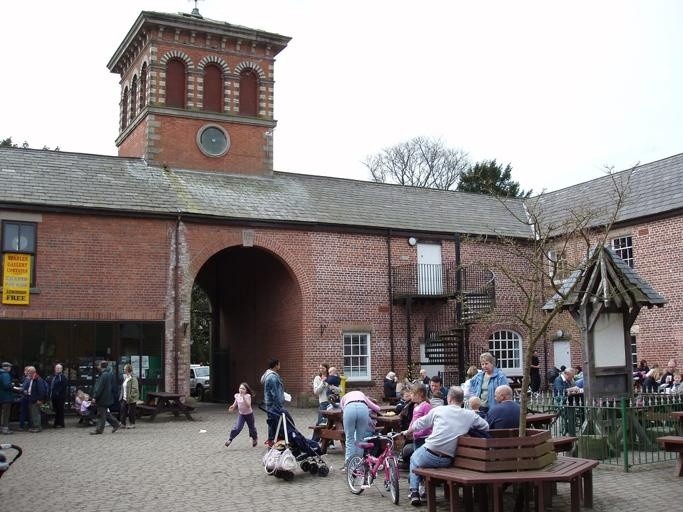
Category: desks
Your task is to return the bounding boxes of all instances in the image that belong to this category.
[505,375,523,383]
[146,391,193,422]
[524,413,556,429]
[669,411,682,477]
[315,405,399,452]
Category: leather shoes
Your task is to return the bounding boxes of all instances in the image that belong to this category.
[1,425,65,434]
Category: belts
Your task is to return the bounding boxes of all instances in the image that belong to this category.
[424,447,450,459]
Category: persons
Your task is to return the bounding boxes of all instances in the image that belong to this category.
[529,349,585,407]
[309,351,521,507]
[633,357,683,394]
[224,382,257,447]
[0,360,140,436]
[260,360,286,443]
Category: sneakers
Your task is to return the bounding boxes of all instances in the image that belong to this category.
[91,431,102,434]
[408,491,426,501]
[121,424,126,428]
[252,436,259,447]
[127,424,136,429]
[111,423,121,433]
[410,491,419,505]
[224,439,232,447]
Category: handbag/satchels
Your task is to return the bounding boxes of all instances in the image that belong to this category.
[263,413,298,475]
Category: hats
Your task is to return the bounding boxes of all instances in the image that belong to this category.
[2,362,13,367]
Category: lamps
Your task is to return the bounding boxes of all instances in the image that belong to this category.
[407,237,416,246]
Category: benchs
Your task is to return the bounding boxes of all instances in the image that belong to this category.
[307,424,327,438]
[508,383,531,391]
[547,433,577,451]
[655,434,682,453]
[414,429,599,511]
[134,405,156,421]
[38,405,58,429]
[177,403,195,414]
[337,425,384,435]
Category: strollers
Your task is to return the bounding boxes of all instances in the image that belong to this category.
[258,402,331,480]
[0,442,22,489]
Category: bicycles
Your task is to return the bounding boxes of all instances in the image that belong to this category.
[342,430,409,506]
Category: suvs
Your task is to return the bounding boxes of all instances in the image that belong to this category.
[189,364,210,401]
